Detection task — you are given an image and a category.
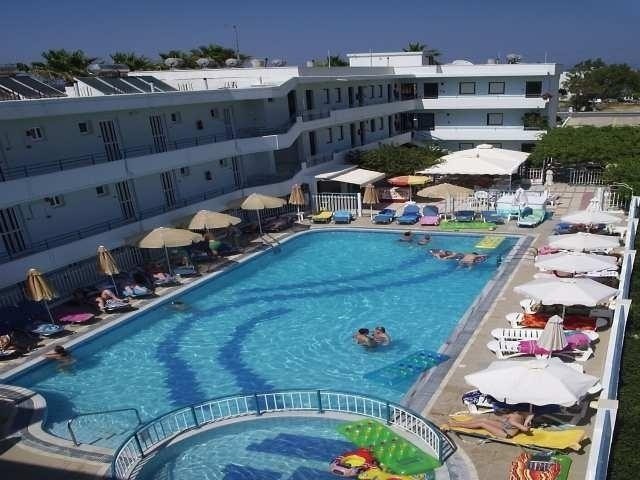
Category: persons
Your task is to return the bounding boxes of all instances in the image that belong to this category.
[356,328,376,347]
[416,234,432,244]
[0,334,32,354]
[400,232,413,242]
[457,251,488,270]
[72,287,129,313]
[373,326,389,347]
[203,232,219,255]
[447,405,536,439]
[44,345,76,374]
[152,272,182,284]
[180,252,195,270]
[430,248,457,260]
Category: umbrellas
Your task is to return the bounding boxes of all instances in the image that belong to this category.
[241,192,288,234]
[287,184,306,222]
[184,209,243,234]
[95,245,122,297]
[544,231,622,251]
[361,183,380,219]
[386,175,431,201]
[22,268,59,325]
[464,356,601,425]
[513,276,620,320]
[558,210,620,230]
[533,249,619,274]
[135,226,205,275]
[535,315,568,359]
[546,170,554,193]
[413,183,472,220]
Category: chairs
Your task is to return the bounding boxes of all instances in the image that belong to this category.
[13,208,352,338]
[445,203,627,457]
[376,202,549,228]
